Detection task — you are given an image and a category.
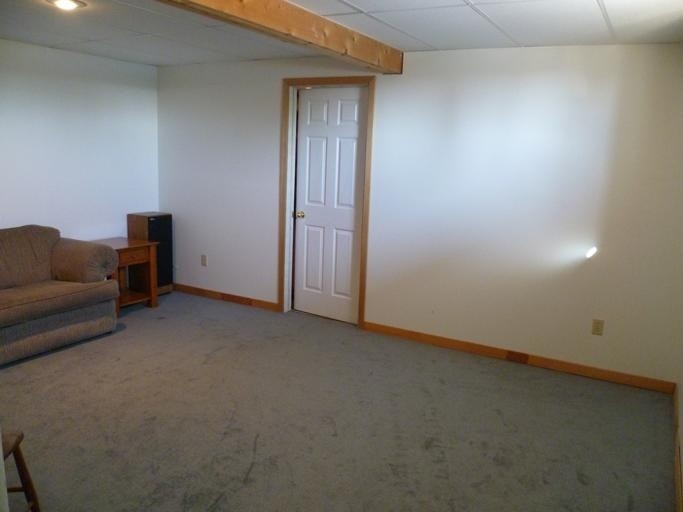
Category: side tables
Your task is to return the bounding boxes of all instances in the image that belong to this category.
[91,237,160,317]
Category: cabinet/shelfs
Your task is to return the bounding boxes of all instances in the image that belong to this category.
[126,212,174,296]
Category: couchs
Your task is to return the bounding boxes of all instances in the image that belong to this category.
[1,225,122,367]
